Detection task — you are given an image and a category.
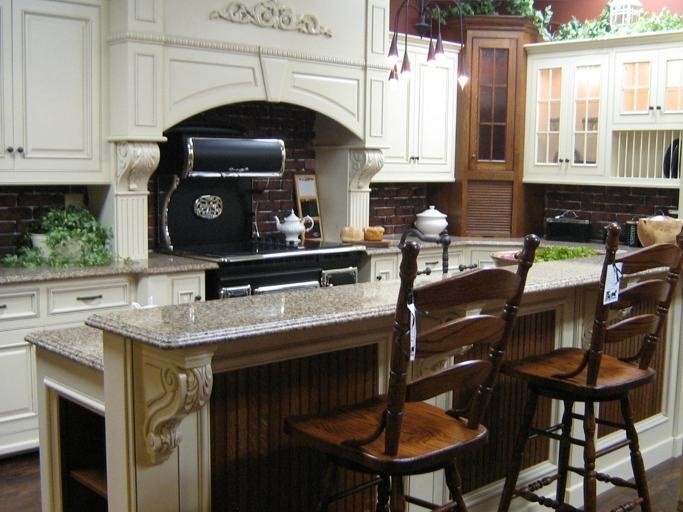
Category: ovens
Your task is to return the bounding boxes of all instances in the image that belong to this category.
[206,244,368,300]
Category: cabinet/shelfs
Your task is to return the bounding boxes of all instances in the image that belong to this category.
[524,28,682,188]
[371,30,466,183]
[0,277,131,456]
[455,15,539,181]
[1,2,110,186]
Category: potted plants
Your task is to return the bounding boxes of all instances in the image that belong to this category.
[1,205,113,273]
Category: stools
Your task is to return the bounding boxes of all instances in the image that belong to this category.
[285,235,541,510]
[498,223,683,511]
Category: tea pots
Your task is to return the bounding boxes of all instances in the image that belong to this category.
[273,208,312,242]
